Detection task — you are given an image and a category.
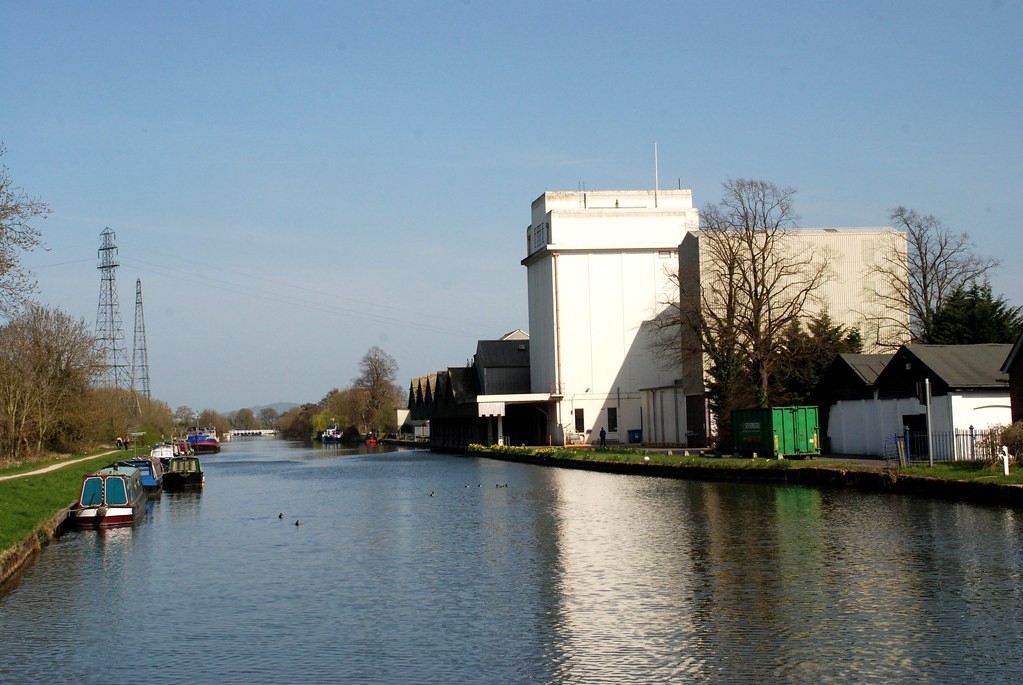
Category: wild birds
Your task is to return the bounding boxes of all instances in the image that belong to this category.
[296,520,301,525]
[465,484,507,488]
[279,512,284,518]
[430,491,435,497]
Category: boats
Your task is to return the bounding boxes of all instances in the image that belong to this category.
[149,441,195,465]
[118,454,165,499]
[163,455,205,490]
[183,416,221,452]
[72,462,149,529]
[322,418,344,443]
[364,432,378,444]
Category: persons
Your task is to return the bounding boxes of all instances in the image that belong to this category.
[123,435,129,450]
[116,436,122,449]
